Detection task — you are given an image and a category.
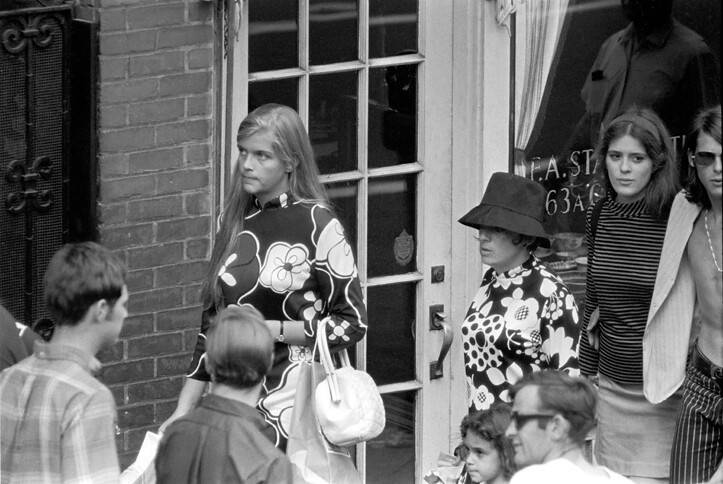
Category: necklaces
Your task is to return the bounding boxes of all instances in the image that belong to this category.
[704,208,723,273]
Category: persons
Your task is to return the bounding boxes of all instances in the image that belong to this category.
[579,103,684,484]
[158,103,367,483]
[544,0,722,234]
[505,369,638,483]
[643,101,723,484]
[456,171,582,418]
[153,310,306,484]
[0,240,130,484]
[453,410,518,484]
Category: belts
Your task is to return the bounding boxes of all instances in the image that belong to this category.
[691,343,723,386]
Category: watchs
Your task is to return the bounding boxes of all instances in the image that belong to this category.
[276,319,285,343]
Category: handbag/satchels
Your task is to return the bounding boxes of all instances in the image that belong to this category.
[286,320,361,484]
[315,317,386,447]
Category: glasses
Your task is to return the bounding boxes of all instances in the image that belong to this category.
[510,411,555,431]
[691,151,723,167]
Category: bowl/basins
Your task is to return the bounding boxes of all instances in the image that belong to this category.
[535,231,586,301]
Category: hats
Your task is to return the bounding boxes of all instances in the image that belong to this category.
[458,172,551,249]
